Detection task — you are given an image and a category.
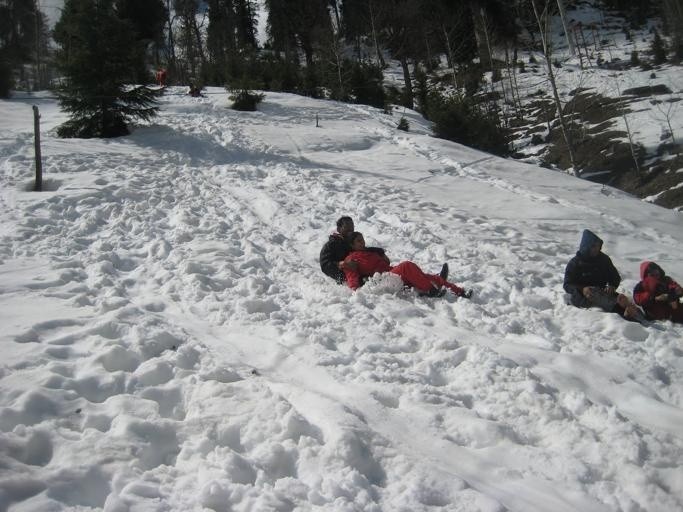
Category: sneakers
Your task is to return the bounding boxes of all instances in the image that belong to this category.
[437,263,473,298]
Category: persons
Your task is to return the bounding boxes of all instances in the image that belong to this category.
[562,229,638,320]
[157,66,166,86]
[319,216,390,282]
[633,261,683,324]
[341,232,473,298]
